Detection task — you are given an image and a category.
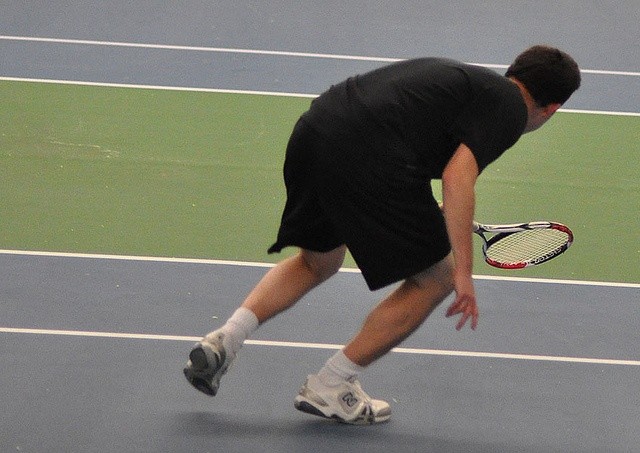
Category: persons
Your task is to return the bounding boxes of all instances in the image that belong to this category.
[183,44,581,425]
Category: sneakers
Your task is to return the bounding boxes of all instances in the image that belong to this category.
[294,373,392,425]
[183,329,236,397]
